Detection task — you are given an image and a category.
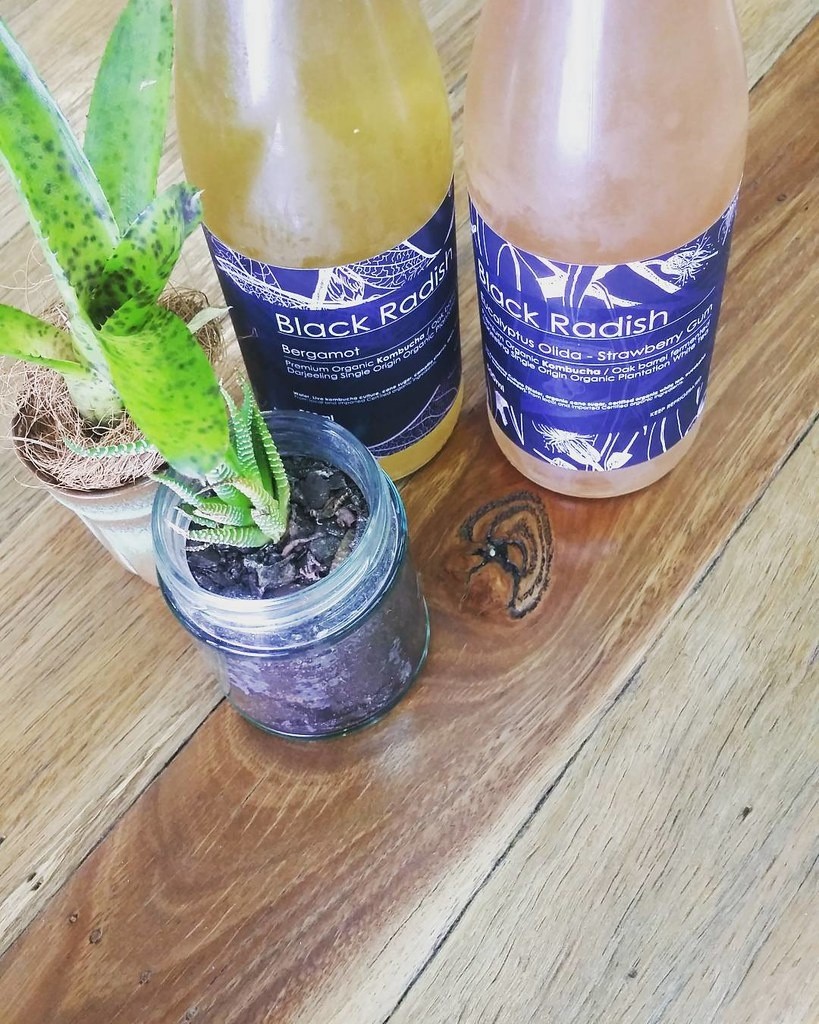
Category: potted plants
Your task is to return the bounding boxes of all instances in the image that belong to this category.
[0,0,432,740]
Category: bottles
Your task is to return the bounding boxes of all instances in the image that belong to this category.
[172,0,455,481]
[464,0,748,498]
